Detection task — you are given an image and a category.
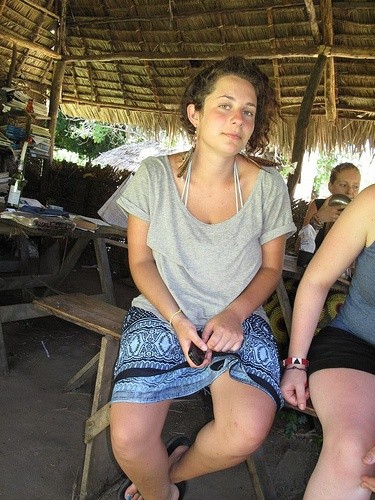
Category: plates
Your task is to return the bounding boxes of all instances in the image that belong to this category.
[284,254,298,262]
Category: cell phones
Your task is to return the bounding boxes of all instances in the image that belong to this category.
[188,331,205,366]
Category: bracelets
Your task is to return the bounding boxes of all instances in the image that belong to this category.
[284,366,308,373]
[282,357,310,368]
[169,308,182,327]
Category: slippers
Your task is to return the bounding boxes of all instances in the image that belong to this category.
[117,434,191,500]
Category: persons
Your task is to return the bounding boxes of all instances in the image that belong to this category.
[279,183,375,500]
[294,162,361,280]
[107,55,298,500]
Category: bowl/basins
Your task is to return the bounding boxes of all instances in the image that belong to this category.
[329,194,351,212]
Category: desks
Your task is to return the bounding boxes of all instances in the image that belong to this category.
[62,225,351,394]
[0,203,120,376]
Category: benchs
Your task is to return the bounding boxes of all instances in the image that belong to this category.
[33,291,316,500]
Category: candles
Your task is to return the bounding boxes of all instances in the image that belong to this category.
[17,141,28,170]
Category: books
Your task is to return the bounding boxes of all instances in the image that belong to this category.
[0,87,51,157]
[0,171,12,193]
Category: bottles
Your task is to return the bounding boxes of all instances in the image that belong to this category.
[6,160,26,209]
